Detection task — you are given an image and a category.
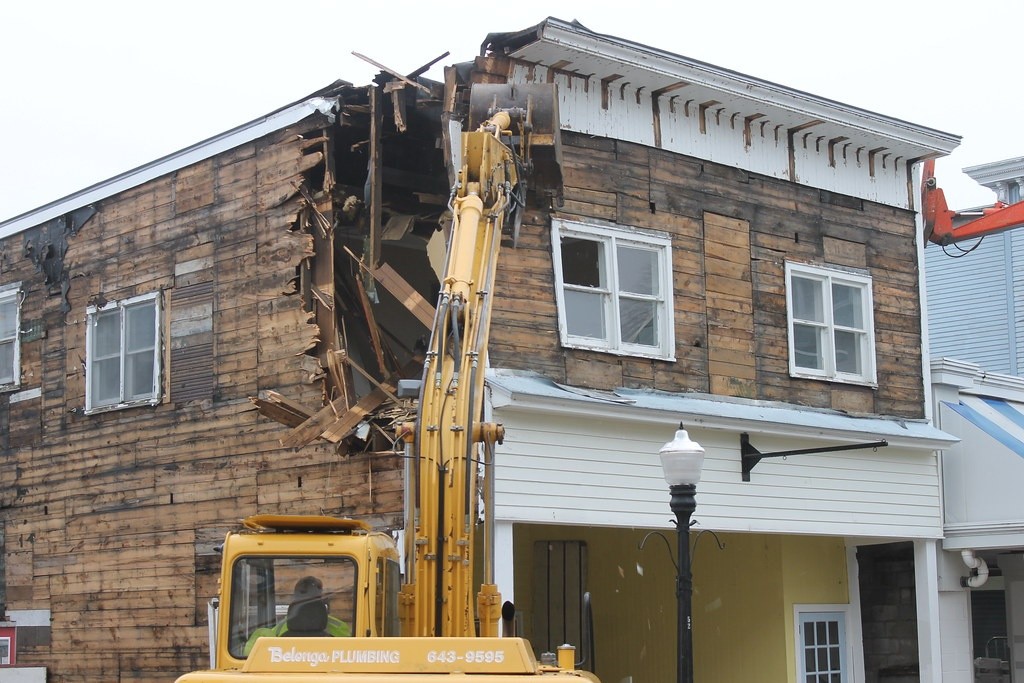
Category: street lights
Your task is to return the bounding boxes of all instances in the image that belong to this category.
[657,420,708,683]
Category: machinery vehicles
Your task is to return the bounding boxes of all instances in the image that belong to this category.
[923,153,1023,245]
[168,85,601,681]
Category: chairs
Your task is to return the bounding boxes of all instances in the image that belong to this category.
[280,601,330,639]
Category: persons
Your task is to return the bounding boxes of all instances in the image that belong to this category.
[244,575,352,655]
